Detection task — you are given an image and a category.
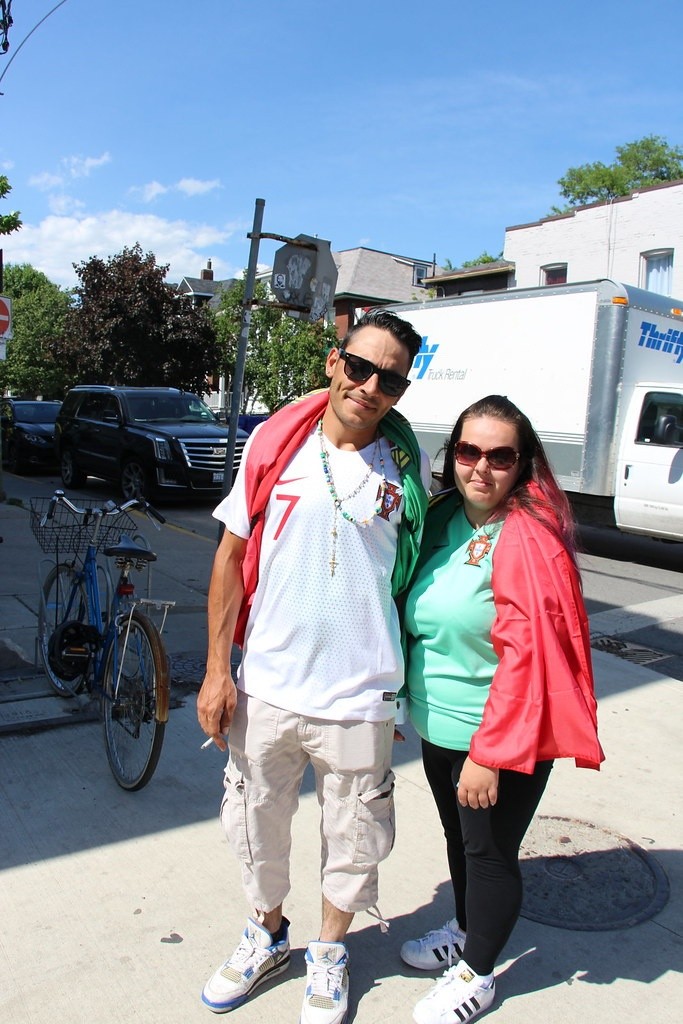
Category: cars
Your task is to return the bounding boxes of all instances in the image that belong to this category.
[0,397,64,474]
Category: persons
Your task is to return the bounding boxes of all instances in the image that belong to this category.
[393,394,604,1024]
[275,275,285,288]
[198,306,432,1024]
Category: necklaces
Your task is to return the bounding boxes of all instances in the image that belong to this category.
[317,418,386,577]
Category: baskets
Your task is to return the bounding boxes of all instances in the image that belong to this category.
[29,495,138,555]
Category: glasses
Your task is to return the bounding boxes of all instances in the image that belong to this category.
[340,351,411,397]
[454,441,521,471]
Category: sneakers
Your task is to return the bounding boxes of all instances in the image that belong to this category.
[412,959,496,1024]
[400,916,468,970]
[200,915,293,1012]
[301,940,349,1024]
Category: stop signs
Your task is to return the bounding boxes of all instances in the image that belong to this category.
[0,295,15,341]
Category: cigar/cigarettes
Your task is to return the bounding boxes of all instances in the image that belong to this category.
[201,737,213,750]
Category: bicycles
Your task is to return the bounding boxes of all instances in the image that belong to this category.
[25,489,176,792]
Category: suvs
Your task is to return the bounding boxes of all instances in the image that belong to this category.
[53,385,250,508]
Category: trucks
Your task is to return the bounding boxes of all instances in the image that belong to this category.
[350,277,683,550]
